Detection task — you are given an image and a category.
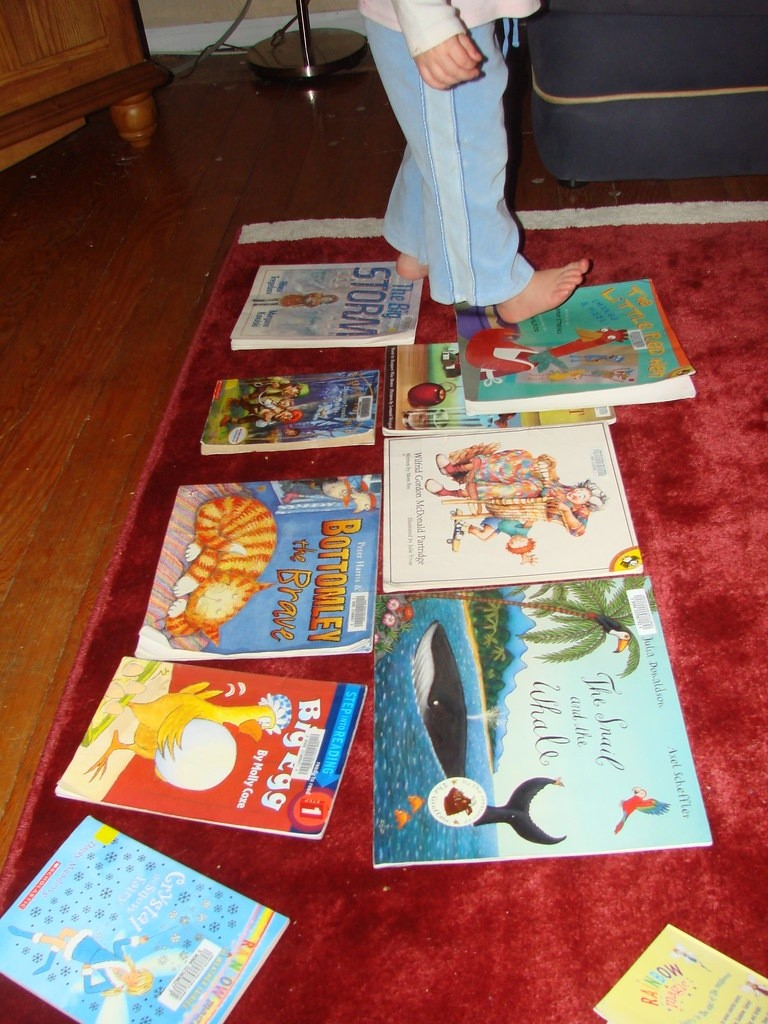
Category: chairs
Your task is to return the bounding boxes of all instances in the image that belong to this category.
[527,1,768,188]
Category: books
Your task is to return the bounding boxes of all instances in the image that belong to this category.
[0,261,768,1024]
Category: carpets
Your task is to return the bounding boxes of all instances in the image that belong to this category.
[0,201,767,1024]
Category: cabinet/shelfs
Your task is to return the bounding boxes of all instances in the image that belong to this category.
[0,0,173,175]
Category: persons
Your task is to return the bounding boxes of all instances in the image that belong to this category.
[357,0,590,324]
